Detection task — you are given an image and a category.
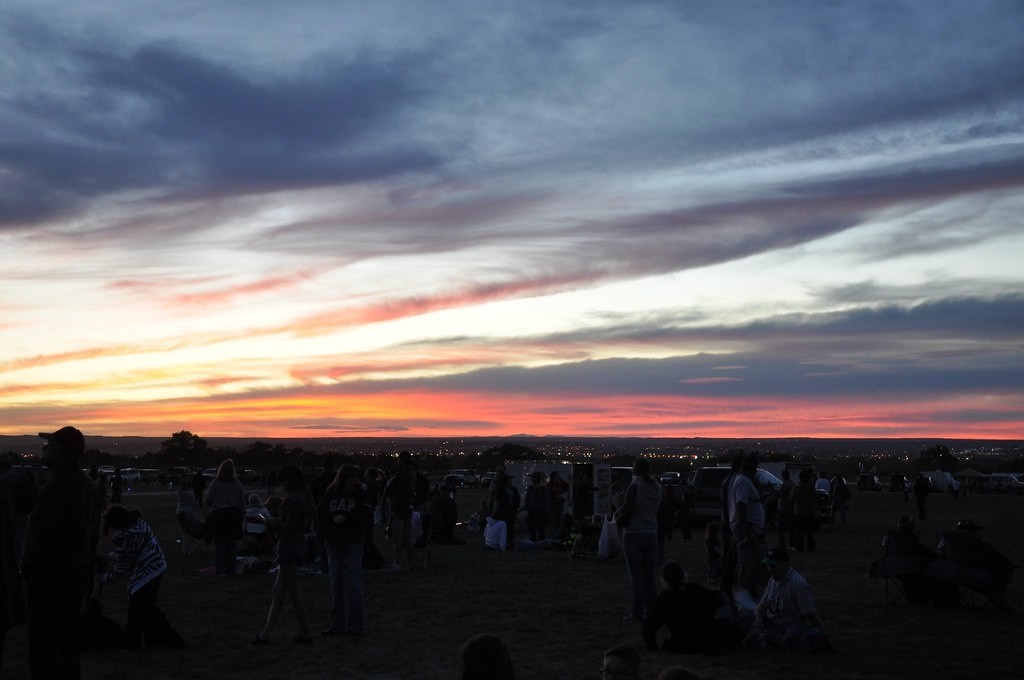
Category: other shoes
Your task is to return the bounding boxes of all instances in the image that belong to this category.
[292,634,314,644]
[252,633,269,646]
[342,631,360,638]
[321,629,343,636]
[622,615,640,625]
[707,577,722,585]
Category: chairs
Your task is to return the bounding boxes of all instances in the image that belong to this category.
[393,510,431,567]
[878,537,913,606]
[937,532,1004,617]
[176,510,211,556]
[577,537,598,560]
[242,514,275,554]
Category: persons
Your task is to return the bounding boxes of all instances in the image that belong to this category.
[600,454,852,680]
[475,467,571,553]
[458,633,516,680]
[171,451,458,645]
[873,471,1015,607]
[0,427,168,680]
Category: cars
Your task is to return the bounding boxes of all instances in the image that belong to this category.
[660,465,732,527]
[480,471,497,488]
[98,464,197,486]
[856,470,1024,495]
[447,468,478,489]
[202,468,217,480]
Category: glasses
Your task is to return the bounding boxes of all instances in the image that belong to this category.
[599,668,639,680]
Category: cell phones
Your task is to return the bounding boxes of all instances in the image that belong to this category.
[259,513,265,519]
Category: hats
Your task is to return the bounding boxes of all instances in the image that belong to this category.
[761,548,790,565]
[951,518,983,534]
[895,515,916,532]
[39,427,86,457]
